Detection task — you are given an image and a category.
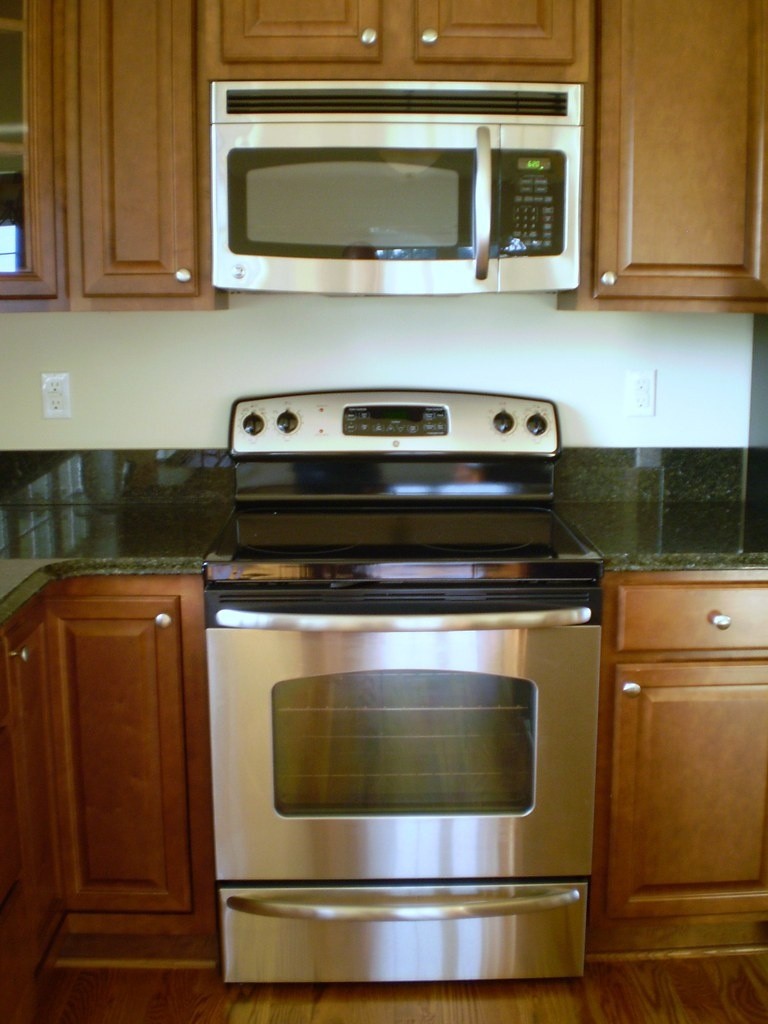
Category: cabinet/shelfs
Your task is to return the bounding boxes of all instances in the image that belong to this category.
[0,453,768,1024]
[0,0,768,315]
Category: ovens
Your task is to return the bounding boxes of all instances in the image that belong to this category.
[204,578,603,995]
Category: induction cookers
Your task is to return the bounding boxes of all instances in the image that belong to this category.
[200,384,603,587]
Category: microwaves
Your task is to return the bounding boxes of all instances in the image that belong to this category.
[207,79,584,299]
